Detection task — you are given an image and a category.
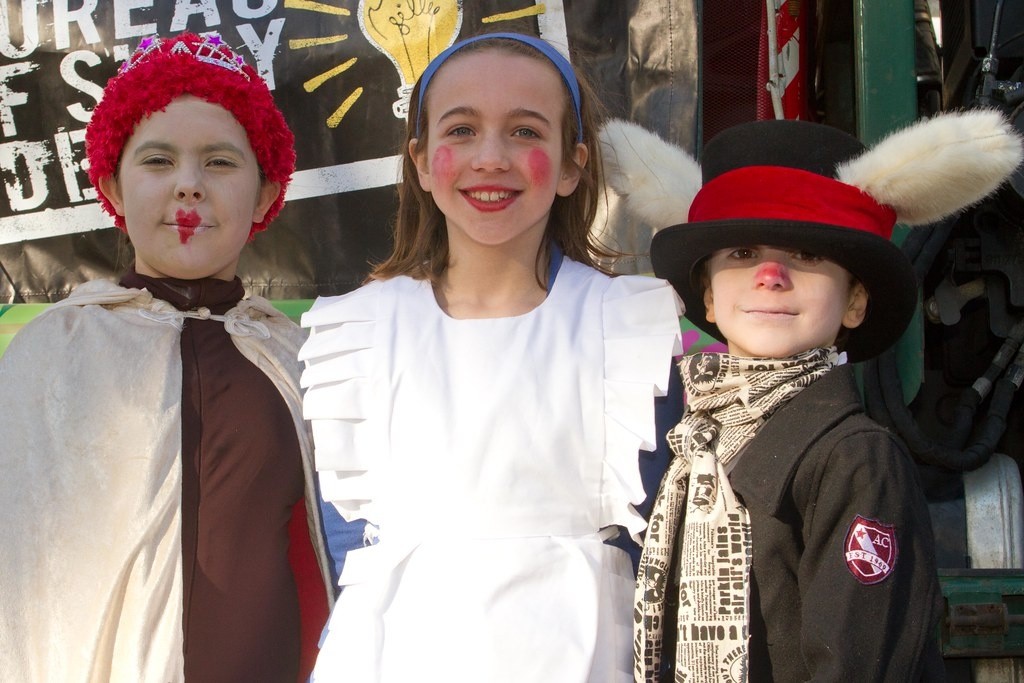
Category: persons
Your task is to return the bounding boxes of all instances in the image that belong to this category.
[0,30,343,683]
[299,32,685,682]
[592,113,1022,683]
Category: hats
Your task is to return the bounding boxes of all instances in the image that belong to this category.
[592,105,1023,362]
[88,31,297,241]
